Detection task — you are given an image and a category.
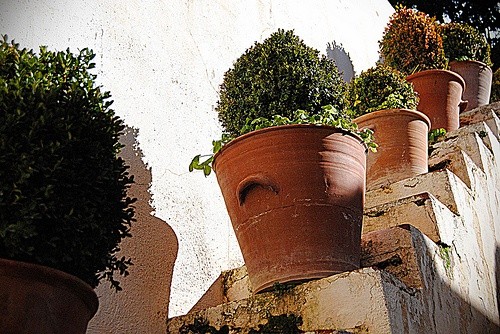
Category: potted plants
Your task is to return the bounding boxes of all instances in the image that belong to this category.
[1,0,493,334]
